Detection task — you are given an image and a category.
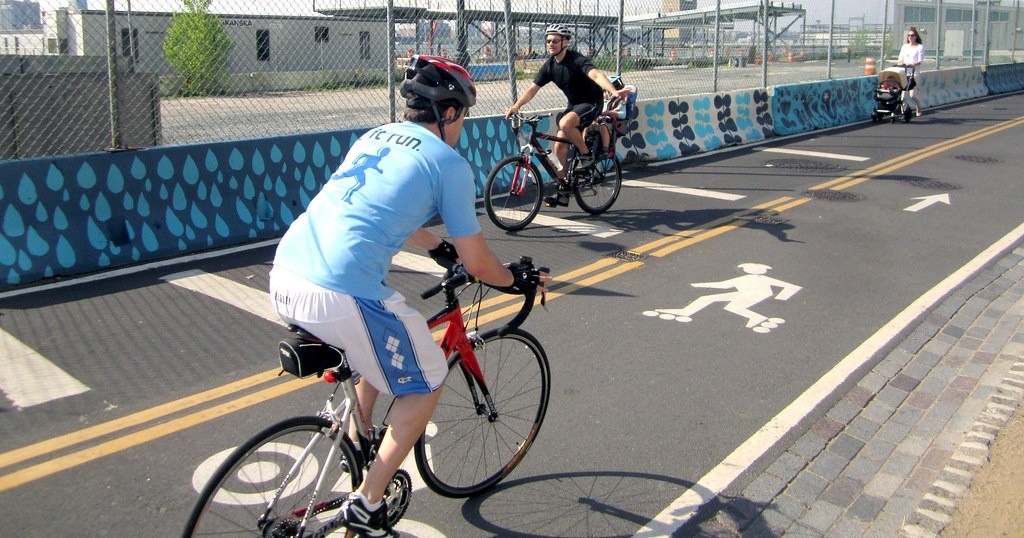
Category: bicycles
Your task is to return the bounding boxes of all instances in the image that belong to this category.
[182,240,551,538]
[483,85,637,232]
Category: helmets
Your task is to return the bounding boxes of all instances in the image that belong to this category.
[545,23,572,36]
[399,55,476,108]
[605,75,624,90]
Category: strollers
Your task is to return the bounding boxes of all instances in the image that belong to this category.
[871,65,916,124]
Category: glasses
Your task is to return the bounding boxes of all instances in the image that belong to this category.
[908,34,917,37]
[546,38,566,44]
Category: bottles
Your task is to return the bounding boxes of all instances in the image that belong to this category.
[546,148,563,171]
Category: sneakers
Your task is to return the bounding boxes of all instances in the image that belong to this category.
[543,191,569,207]
[575,149,595,171]
[335,493,400,538]
[340,426,381,473]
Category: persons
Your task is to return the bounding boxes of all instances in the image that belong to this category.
[267,53,552,538]
[505,23,630,182]
[581,76,629,160]
[897,27,924,118]
[880,82,889,90]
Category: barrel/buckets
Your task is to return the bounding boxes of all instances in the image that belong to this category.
[734,59,739,67]
[738,57,746,68]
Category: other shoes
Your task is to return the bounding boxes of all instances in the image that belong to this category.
[599,148,609,159]
[899,115,904,119]
[916,105,922,117]
